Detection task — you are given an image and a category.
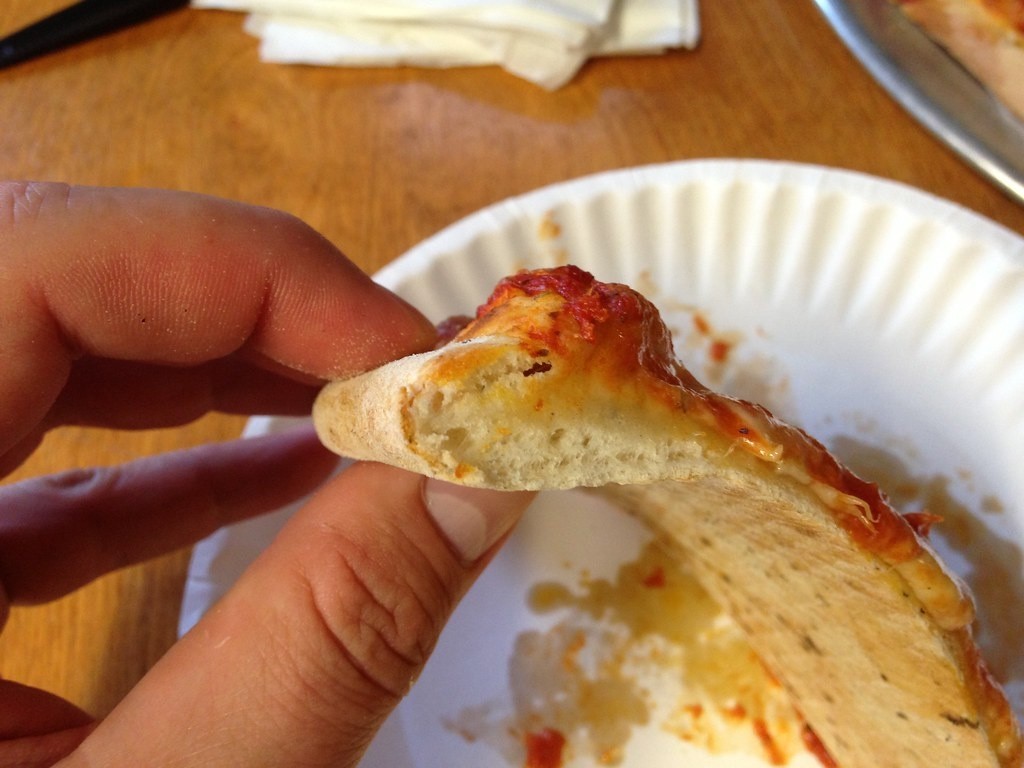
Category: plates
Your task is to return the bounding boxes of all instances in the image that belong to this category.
[174,157,1024,768]
[810,0,1024,208]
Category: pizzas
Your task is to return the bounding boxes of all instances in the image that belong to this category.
[312,266,1024,768]
[894,0,1024,127]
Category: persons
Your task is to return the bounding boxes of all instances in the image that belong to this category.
[2,180,540,768]
